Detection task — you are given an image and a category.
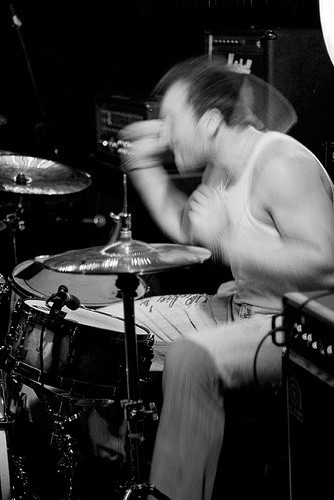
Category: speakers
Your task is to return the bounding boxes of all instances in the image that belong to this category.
[204,28,334,160]
[276,353,333,500]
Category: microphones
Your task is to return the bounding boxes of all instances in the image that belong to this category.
[56,216,106,227]
[55,292,80,310]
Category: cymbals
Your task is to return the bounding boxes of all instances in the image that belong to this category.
[48,237,215,274]
[0,153,91,194]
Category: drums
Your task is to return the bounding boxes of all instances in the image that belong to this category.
[14,298,153,403]
[4,258,151,359]
